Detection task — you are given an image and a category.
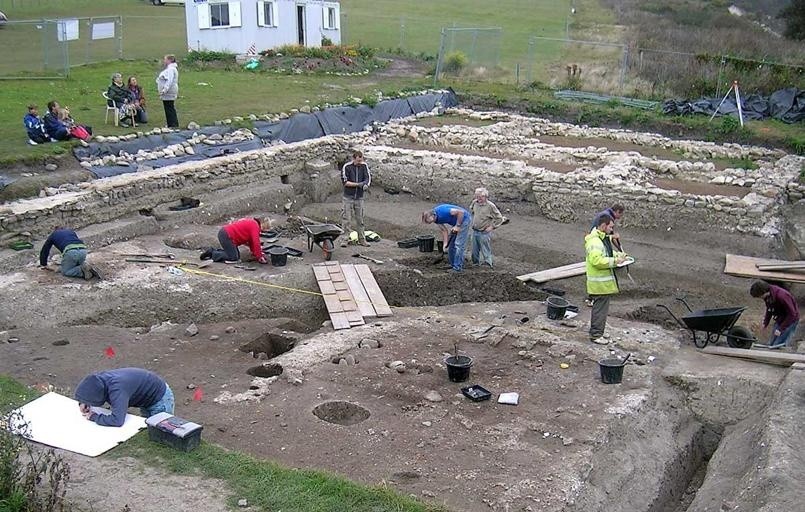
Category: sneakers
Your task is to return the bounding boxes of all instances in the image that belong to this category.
[200,249,213,260]
[591,332,610,345]
[82,264,104,280]
[357,239,371,247]
[340,239,350,246]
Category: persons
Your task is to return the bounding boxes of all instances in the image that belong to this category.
[108,73,139,128]
[24,103,51,144]
[469,188,504,268]
[58,109,93,142]
[585,203,625,307]
[128,76,148,123]
[74,367,175,427]
[750,280,800,349]
[341,151,372,247]
[422,204,471,273]
[155,54,179,127]
[585,215,625,344]
[42,101,70,140]
[200,217,267,264]
[40,226,104,281]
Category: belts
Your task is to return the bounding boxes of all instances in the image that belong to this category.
[65,247,85,252]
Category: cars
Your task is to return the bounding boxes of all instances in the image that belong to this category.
[148,1,185,7]
[0,12,7,26]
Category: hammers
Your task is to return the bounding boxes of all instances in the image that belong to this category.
[121,248,176,260]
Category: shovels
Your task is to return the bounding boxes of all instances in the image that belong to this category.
[482,317,505,335]
[753,342,785,349]
[616,238,635,283]
[432,230,457,265]
[351,253,384,264]
[266,231,282,242]
[125,258,214,268]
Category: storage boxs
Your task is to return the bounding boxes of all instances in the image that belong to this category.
[143,411,205,451]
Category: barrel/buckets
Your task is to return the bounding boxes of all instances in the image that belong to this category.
[269,247,289,267]
[437,240,443,252]
[415,234,435,252]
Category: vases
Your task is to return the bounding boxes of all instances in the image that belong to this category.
[418,234,435,251]
[270,246,288,265]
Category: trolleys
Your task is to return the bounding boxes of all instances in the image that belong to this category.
[657,295,759,350]
[298,215,344,261]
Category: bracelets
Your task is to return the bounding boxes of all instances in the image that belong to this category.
[456,224,461,227]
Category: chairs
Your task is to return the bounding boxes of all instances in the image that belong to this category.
[103,90,121,126]
